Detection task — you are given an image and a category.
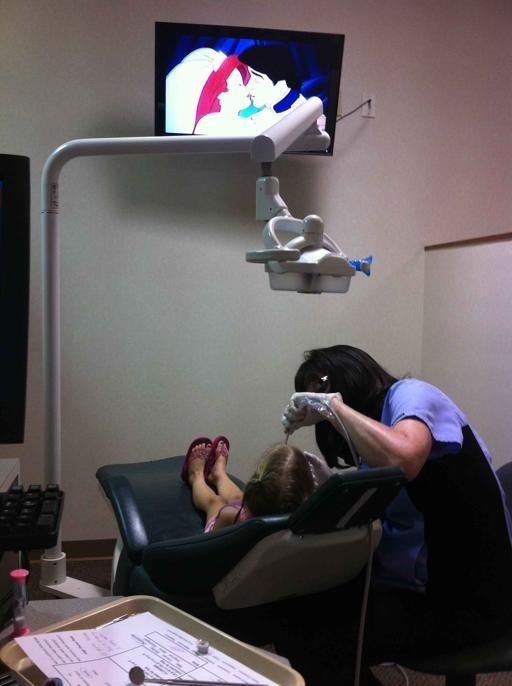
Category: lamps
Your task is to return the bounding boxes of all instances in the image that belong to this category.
[42,95,372,595]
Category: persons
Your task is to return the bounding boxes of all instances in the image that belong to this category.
[181,435,314,534]
[270,345,512,686]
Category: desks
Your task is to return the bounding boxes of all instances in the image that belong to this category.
[1,597,292,685]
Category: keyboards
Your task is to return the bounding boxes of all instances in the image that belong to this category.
[0,483,66,551]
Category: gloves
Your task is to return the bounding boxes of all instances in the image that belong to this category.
[281,391,344,436]
[303,450,340,486]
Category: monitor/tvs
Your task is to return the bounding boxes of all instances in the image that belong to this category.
[154,20,348,157]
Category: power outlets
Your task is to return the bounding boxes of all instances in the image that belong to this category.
[359,91,378,116]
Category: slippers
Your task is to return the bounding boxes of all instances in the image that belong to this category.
[180,435,213,483]
[204,436,230,482]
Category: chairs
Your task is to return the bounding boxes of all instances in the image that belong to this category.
[95,455,408,645]
